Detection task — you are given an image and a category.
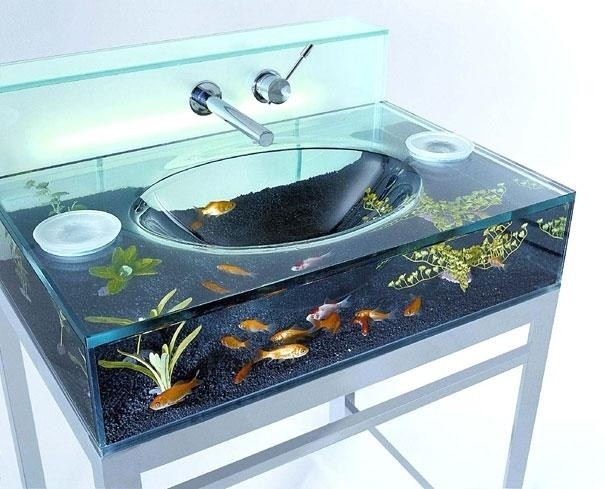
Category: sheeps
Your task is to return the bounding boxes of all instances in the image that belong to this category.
[189,79,273,149]
